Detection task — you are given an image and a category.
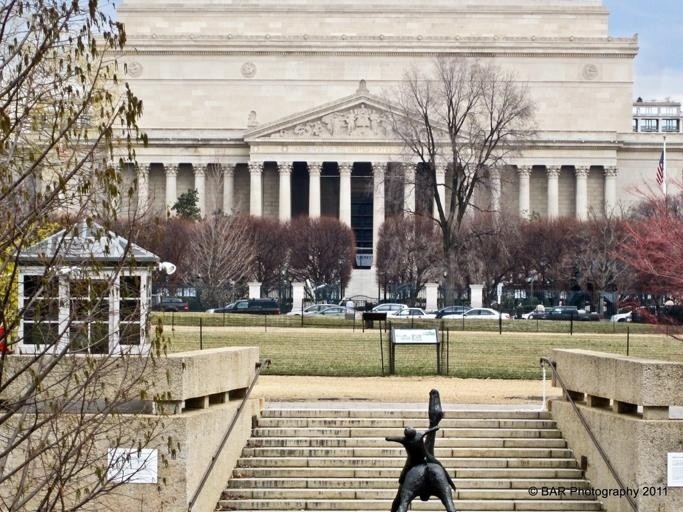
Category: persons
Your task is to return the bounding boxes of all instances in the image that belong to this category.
[278,102,401,138]
[383,422,456,511]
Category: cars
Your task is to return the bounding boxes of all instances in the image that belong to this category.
[151,296,190,313]
[204,297,673,324]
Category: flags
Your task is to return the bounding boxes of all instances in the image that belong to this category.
[654,147,665,193]
